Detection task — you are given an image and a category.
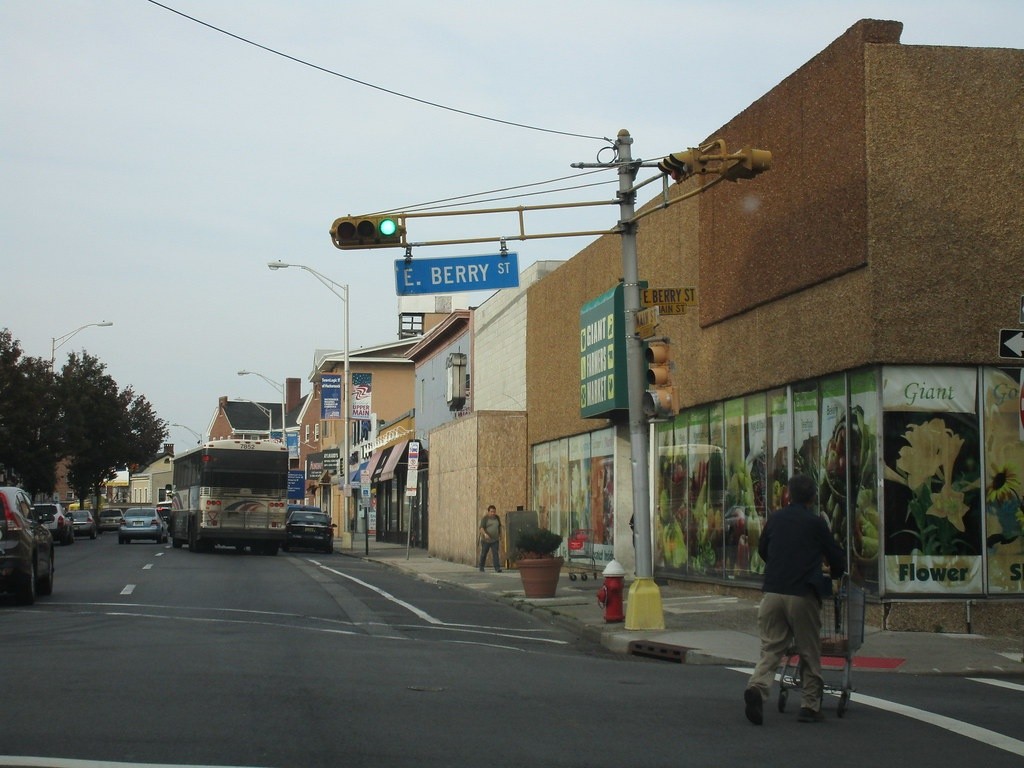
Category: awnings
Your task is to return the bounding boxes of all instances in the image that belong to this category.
[381,440,409,480]
[364,445,394,483]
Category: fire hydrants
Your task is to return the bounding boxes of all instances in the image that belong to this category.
[596,558,625,623]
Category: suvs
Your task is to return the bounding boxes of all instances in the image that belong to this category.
[0,488,74,604]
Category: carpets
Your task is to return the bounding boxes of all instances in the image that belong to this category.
[777,648,903,669]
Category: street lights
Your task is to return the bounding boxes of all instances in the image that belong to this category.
[50,321,113,373]
[268,260,353,549]
[235,397,272,439]
[237,370,286,445]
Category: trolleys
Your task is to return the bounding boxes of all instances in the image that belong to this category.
[567,529,598,581]
[778,572,866,717]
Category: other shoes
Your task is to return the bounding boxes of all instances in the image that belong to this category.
[798,708,825,722]
[480,568,484,571]
[746,687,763,725]
[495,568,502,572]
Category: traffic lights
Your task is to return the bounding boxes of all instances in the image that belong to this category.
[657,147,703,183]
[726,144,772,180]
[643,345,674,419]
[330,214,401,249]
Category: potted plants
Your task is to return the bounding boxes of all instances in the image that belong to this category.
[511,524,564,597]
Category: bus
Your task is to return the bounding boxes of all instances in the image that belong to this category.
[166,439,289,555]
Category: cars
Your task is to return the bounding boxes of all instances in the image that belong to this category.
[156,501,172,523]
[281,505,337,554]
[97,509,124,534]
[66,511,97,540]
[118,508,169,544]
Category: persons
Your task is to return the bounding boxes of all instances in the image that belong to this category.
[629,513,635,576]
[743,473,846,725]
[479,505,504,572]
